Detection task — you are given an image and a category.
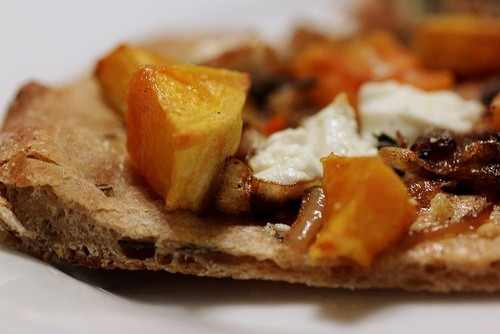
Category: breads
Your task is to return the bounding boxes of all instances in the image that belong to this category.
[0,76,499,293]
